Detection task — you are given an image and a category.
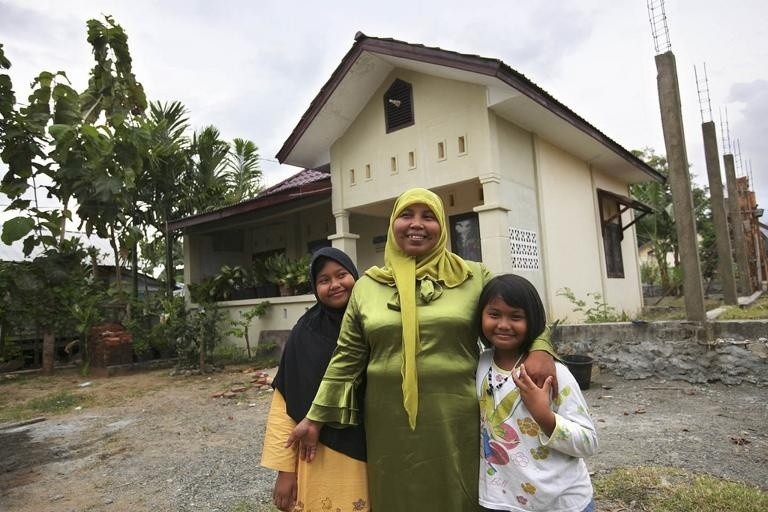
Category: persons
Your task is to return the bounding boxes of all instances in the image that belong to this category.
[260,246,368,512]
[284,188,557,511]
[477,274,596,512]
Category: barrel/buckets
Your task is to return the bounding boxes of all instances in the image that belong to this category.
[91,324,123,367]
[103,332,132,366]
[561,355,594,390]
